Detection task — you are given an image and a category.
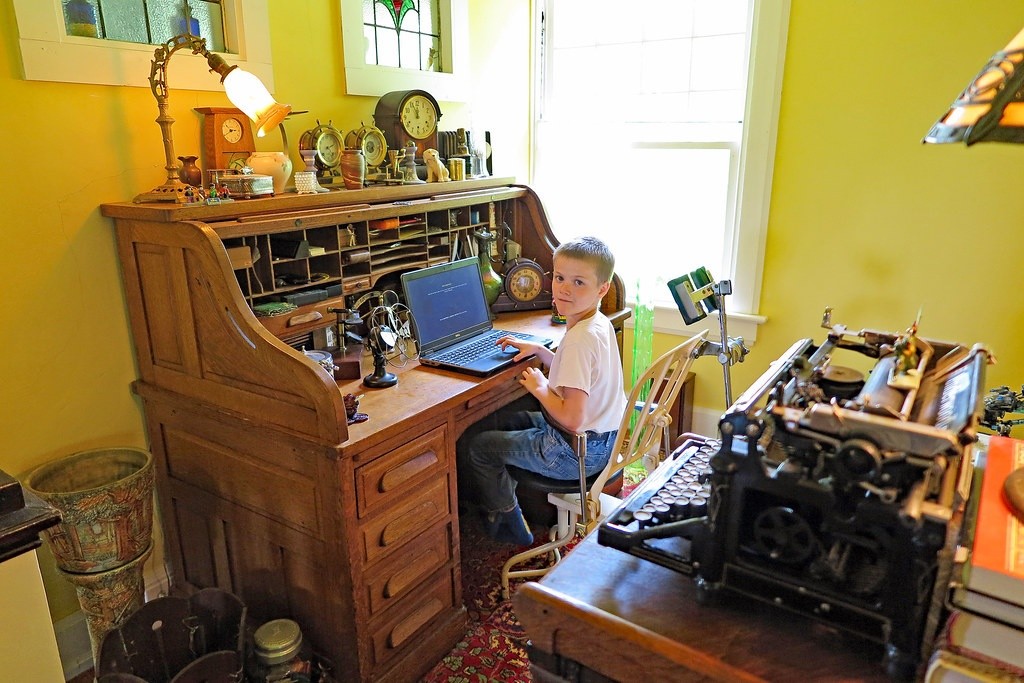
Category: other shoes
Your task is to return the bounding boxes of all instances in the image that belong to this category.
[516,495,557,527]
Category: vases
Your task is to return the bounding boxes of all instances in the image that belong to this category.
[341,149,365,190]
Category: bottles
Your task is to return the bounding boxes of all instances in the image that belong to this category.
[244,619,334,683]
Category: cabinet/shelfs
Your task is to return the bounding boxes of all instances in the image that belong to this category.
[214,191,518,351]
[646,368,696,455]
[136,391,477,682]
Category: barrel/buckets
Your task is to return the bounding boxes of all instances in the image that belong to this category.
[19,446,154,573]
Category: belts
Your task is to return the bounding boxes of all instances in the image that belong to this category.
[585,430,618,440]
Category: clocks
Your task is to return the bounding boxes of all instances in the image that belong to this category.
[194,106,256,177]
[373,90,443,164]
[492,253,553,313]
[299,118,345,183]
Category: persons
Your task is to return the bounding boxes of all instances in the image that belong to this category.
[456,233,631,547]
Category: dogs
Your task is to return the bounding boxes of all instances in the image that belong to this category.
[423,148,452,182]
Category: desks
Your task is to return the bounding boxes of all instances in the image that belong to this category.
[130,306,632,461]
[512,431,899,683]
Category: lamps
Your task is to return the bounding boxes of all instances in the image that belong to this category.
[132,33,293,205]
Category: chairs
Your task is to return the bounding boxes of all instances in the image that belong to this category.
[501,328,708,600]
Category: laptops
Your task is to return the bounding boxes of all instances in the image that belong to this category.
[401,256,553,379]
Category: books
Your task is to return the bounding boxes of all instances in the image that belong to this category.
[916,434,1024,683]
